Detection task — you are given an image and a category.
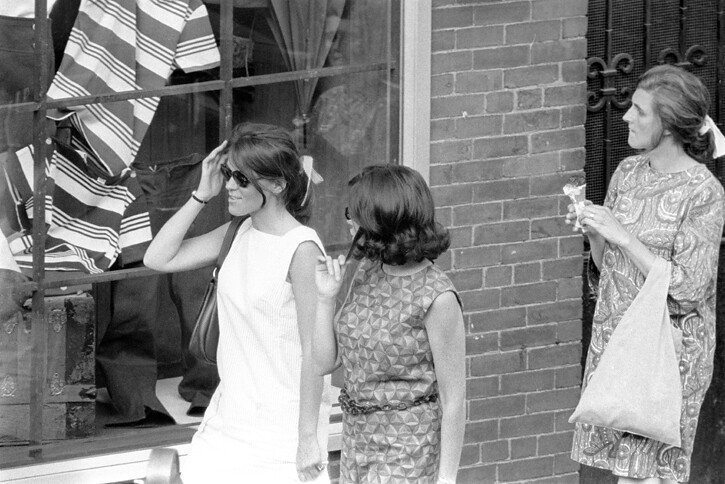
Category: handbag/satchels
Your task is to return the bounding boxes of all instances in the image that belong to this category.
[189,215,253,365]
[567,257,685,448]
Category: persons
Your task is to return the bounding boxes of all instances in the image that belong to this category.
[566,64,725,484]
[144,123,326,484]
[316,164,466,484]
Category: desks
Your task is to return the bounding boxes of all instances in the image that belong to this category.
[154,376,347,425]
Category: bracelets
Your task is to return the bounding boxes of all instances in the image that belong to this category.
[192,194,207,204]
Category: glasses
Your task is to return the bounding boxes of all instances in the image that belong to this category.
[344,208,351,220]
[220,164,269,189]
